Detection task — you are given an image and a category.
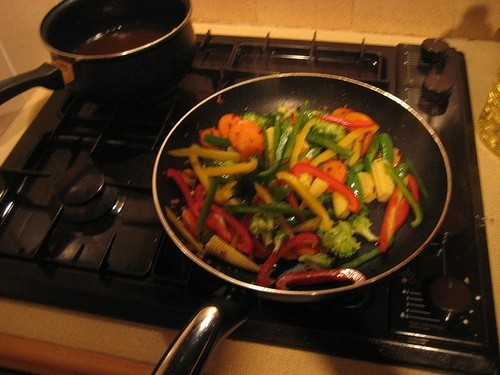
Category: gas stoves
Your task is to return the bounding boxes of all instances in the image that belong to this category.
[5,33,500,375]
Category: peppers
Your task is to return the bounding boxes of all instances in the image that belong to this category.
[165,108,430,291]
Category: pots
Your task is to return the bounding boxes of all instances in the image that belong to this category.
[149,73,451,375]
[0,0,196,108]
